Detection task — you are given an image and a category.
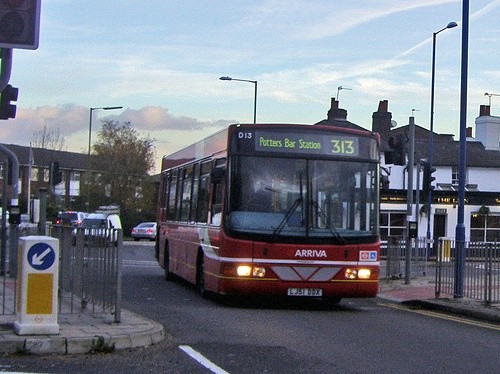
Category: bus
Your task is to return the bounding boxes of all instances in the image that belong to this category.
[155,123,389,307]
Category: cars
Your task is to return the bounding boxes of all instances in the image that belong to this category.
[55,211,86,227]
[72,213,123,247]
[130,222,157,241]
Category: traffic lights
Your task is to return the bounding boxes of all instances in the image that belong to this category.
[388,135,404,166]
[0,84,19,119]
[424,167,436,189]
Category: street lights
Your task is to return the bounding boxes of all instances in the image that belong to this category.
[85,105,123,214]
[218,76,257,124]
[425,21,458,258]
